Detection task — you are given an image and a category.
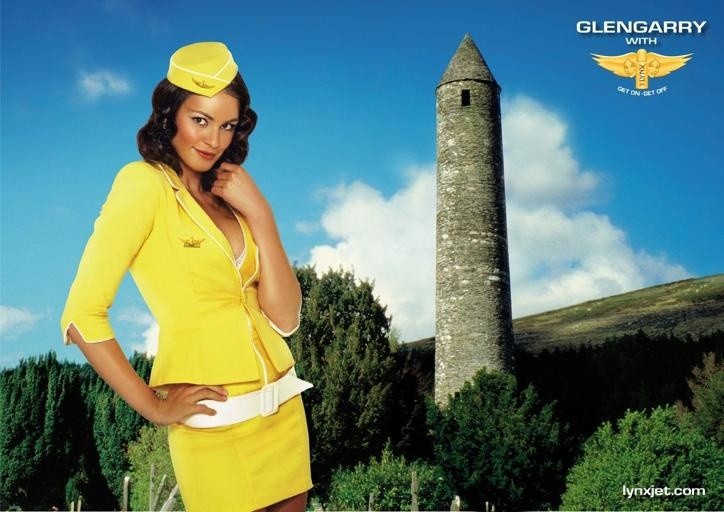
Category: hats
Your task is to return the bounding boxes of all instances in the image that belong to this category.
[167,38,240,98]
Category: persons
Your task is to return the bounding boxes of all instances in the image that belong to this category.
[60,41,314,511]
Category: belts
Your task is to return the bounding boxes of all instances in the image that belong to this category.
[177,366,317,431]
